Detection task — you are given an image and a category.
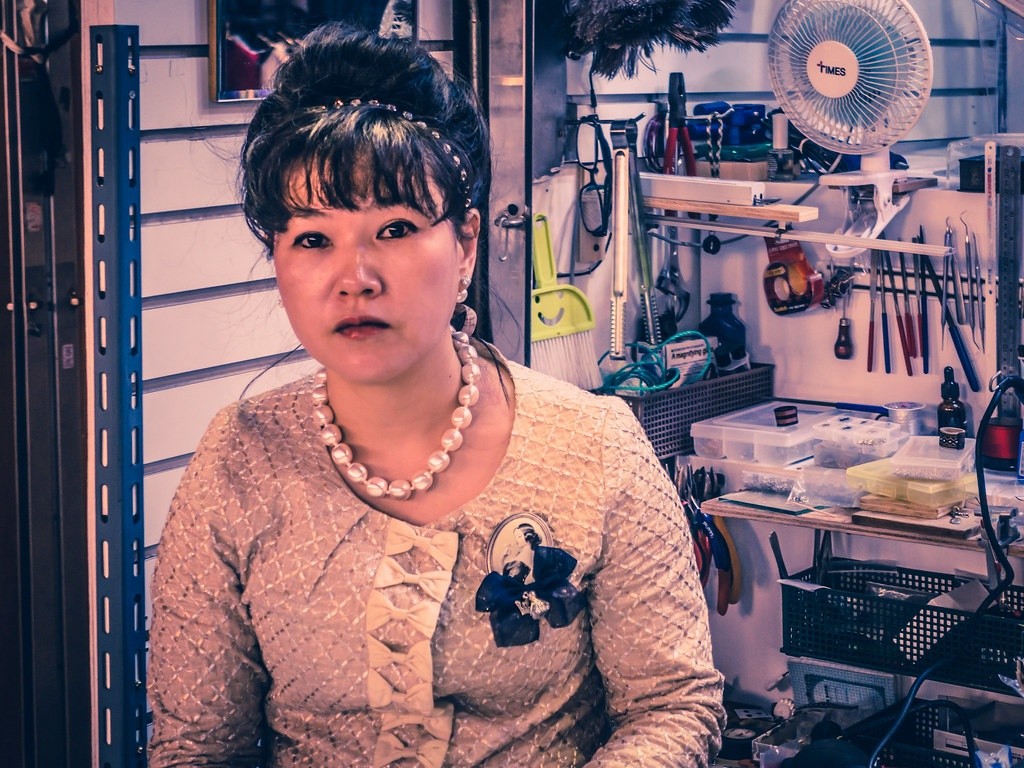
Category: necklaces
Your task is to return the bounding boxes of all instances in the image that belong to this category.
[310,326,481,502]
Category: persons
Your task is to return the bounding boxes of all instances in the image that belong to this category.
[143,23,727,768]
[501,524,543,586]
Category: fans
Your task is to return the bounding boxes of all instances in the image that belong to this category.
[767,1,933,258]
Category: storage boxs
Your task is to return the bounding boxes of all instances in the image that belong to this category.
[751,698,1024,768]
[688,398,978,518]
[776,557,1023,699]
[607,359,776,461]
